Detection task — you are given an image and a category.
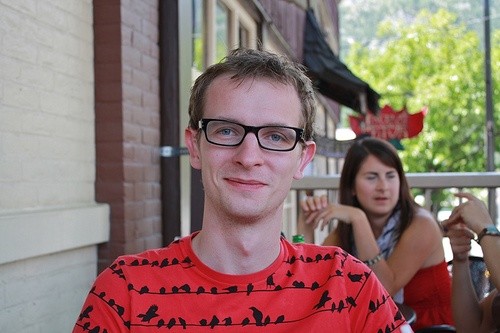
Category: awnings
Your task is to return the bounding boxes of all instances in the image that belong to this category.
[303,8,381,116]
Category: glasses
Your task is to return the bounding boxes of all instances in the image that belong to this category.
[196,118,307,152]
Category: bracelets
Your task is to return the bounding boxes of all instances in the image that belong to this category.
[364,252,382,266]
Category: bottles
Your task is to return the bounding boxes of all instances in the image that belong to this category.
[292,235,305,242]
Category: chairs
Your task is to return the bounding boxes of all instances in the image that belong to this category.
[447,256,494,302]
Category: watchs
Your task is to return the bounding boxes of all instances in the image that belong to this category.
[478,225,500,242]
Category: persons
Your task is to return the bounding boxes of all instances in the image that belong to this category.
[301,137,455,330]
[441,192,500,333]
[72,43,413,333]
